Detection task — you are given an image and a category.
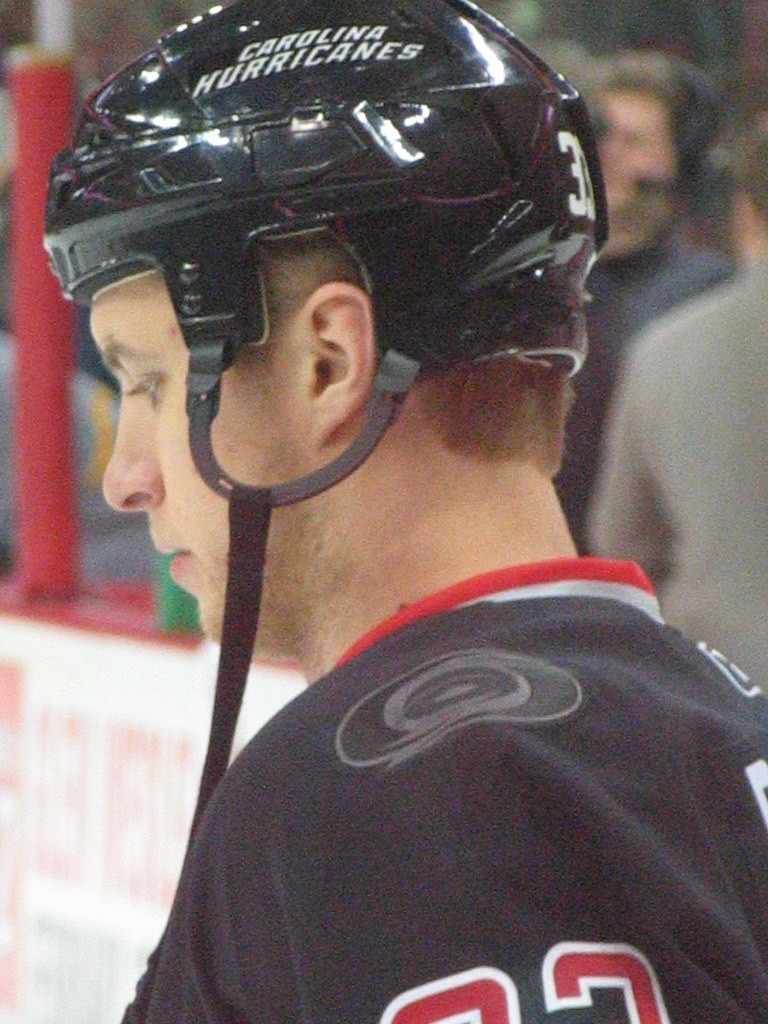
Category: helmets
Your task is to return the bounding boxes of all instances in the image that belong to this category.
[41,0,610,378]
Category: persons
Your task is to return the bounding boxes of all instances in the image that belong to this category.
[552,47,732,555]
[44,2,768,1024]
[592,120,768,688]
